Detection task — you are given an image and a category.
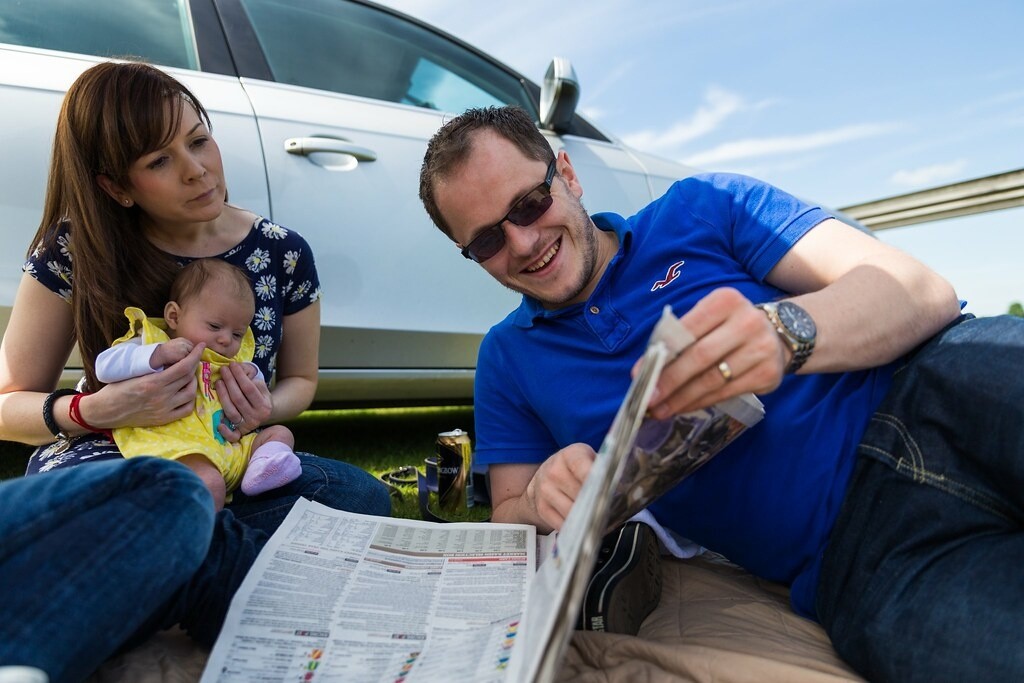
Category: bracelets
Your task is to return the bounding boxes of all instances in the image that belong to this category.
[68,393,112,442]
[42,388,80,441]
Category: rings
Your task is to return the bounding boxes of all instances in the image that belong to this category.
[230,418,244,430]
[717,362,732,380]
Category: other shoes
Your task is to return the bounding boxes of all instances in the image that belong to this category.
[574,521,663,635]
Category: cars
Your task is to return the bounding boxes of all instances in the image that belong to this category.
[0,2,710,426]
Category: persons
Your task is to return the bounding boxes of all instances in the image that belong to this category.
[94,259,311,511]
[419,106,1023,682]
[0,61,393,682]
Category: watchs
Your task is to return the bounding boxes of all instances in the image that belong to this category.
[756,301,817,376]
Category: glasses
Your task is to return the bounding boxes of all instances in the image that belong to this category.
[461,160,556,263]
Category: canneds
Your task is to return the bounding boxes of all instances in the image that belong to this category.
[435,429,474,515]
